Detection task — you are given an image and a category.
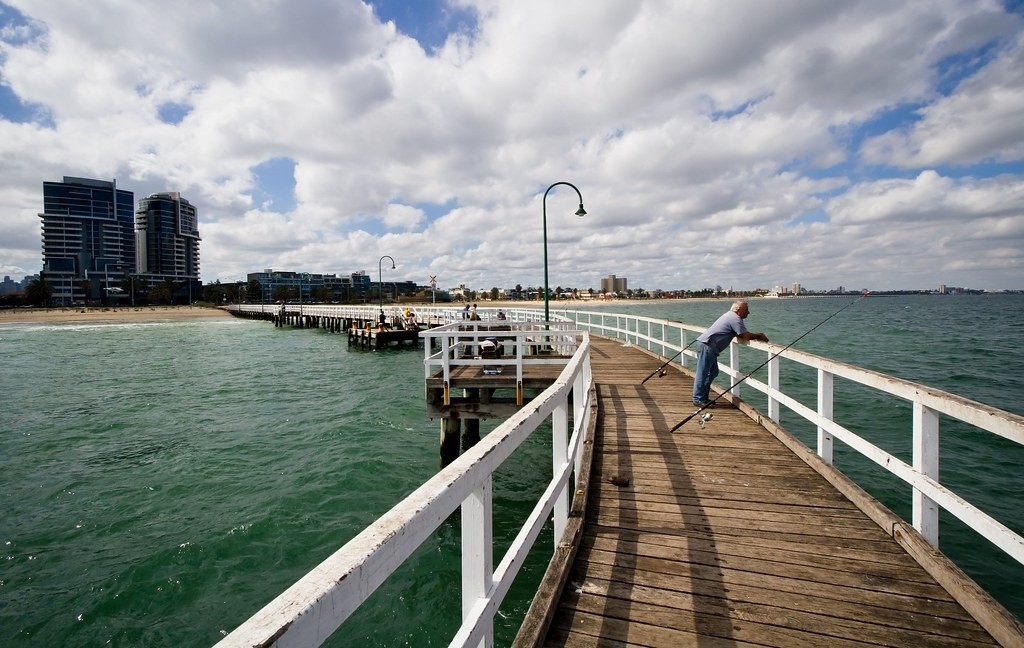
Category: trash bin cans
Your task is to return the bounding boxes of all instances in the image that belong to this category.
[481,337,503,374]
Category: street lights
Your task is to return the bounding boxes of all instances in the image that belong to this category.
[379,255,396,307]
[300,272,311,314]
[542,181,588,350]
[262,279,272,313]
[239,286,247,313]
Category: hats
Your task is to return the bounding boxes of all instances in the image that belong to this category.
[498,309,503,313]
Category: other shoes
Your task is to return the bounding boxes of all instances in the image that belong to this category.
[703,399,716,405]
[693,401,715,409]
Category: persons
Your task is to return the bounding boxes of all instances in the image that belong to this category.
[497,309,506,320]
[407,313,418,325]
[281,303,285,309]
[462,304,470,318]
[693,301,769,408]
[470,304,481,321]
[404,308,410,317]
[380,311,389,332]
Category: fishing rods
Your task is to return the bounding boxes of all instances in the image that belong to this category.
[640,339,696,385]
[669,291,869,433]
